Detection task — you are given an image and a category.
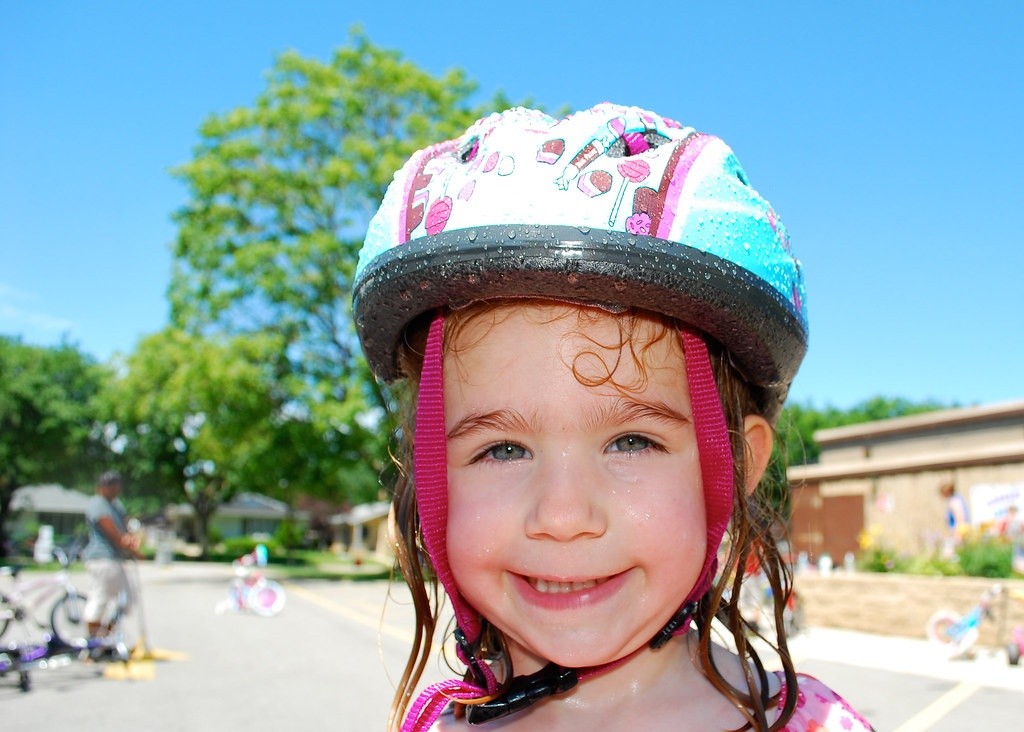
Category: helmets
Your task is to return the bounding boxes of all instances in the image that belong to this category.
[350,102,810,428]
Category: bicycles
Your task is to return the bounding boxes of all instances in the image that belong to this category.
[228,544,285,617]
[927,584,1004,661]
[0,635,128,692]
[0,546,110,646]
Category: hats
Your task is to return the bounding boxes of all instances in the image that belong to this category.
[99,470,122,483]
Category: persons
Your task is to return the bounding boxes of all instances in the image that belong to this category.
[228,543,268,609]
[724,534,763,636]
[79,466,142,665]
[351,101,879,732]
[938,481,967,575]
